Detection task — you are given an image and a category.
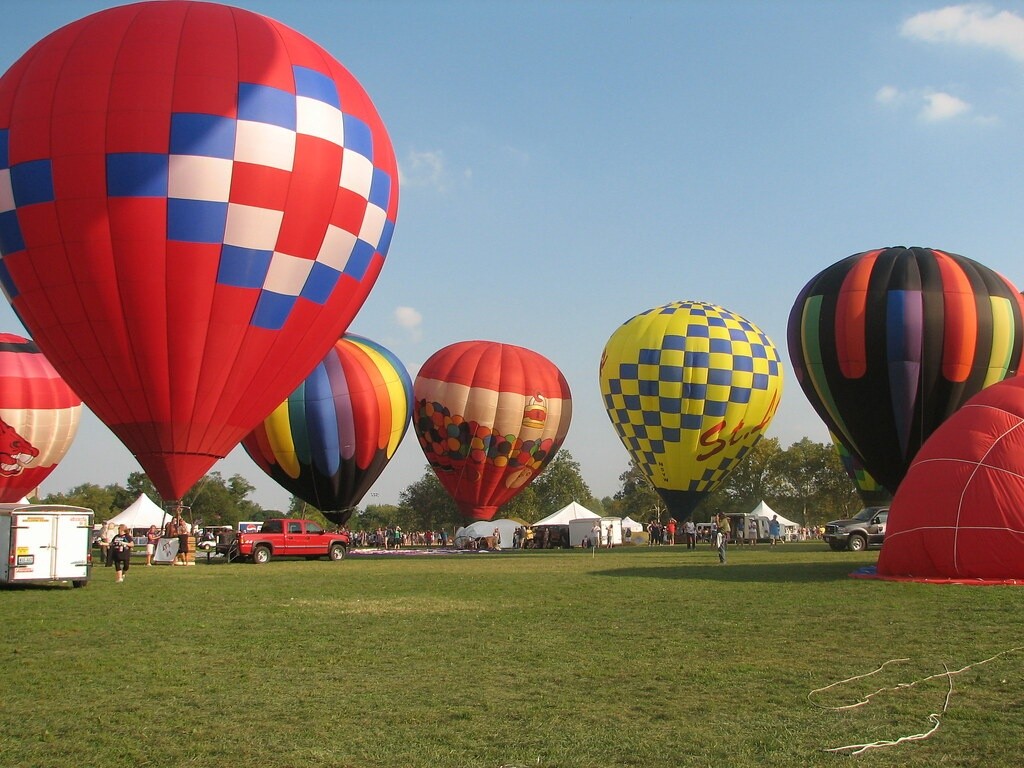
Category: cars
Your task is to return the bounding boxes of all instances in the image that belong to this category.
[198,540,217,549]
[473,536,501,549]
[197,529,204,537]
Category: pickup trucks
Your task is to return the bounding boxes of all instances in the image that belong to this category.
[215,519,349,564]
[821,506,890,551]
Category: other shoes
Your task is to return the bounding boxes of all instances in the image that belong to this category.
[100,560,106,563]
[146,563,152,566]
[105,565,113,567]
[719,560,727,564]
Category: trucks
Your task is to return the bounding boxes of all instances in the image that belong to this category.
[93,523,103,548]
[0,503,95,588]
[696,513,770,544]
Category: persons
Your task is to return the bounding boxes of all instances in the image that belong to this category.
[798,524,826,540]
[329,526,449,550]
[493,526,501,551]
[625,528,632,544]
[110,523,133,583]
[769,515,780,549]
[543,527,550,549]
[736,517,745,549]
[99,521,115,567]
[606,524,613,549]
[591,523,600,548]
[144,516,188,567]
[748,517,757,548]
[716,511,731,565]
[205,529,229,541]
[513,526,534,549]
[647,517,717,549]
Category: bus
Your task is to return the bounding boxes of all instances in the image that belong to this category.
[238,522,264,534]
[203,525,233,538]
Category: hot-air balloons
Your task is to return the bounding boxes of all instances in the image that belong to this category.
[0,331,83,501]
[786,244,1024,505]
[0,1,399,501]
[847,375,1024,585]
[599,299,785,519]
[413,339,573,526]
[241,330,413,529]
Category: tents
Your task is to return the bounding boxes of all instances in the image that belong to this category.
[530,502,602,526]
[750,499,800,527]
[621,515,643,532]
[93,493,199,530]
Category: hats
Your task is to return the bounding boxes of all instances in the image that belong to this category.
[102,520,107,525]
[749,518,754,521]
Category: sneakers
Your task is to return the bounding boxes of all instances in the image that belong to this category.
[115,575,126,584]
